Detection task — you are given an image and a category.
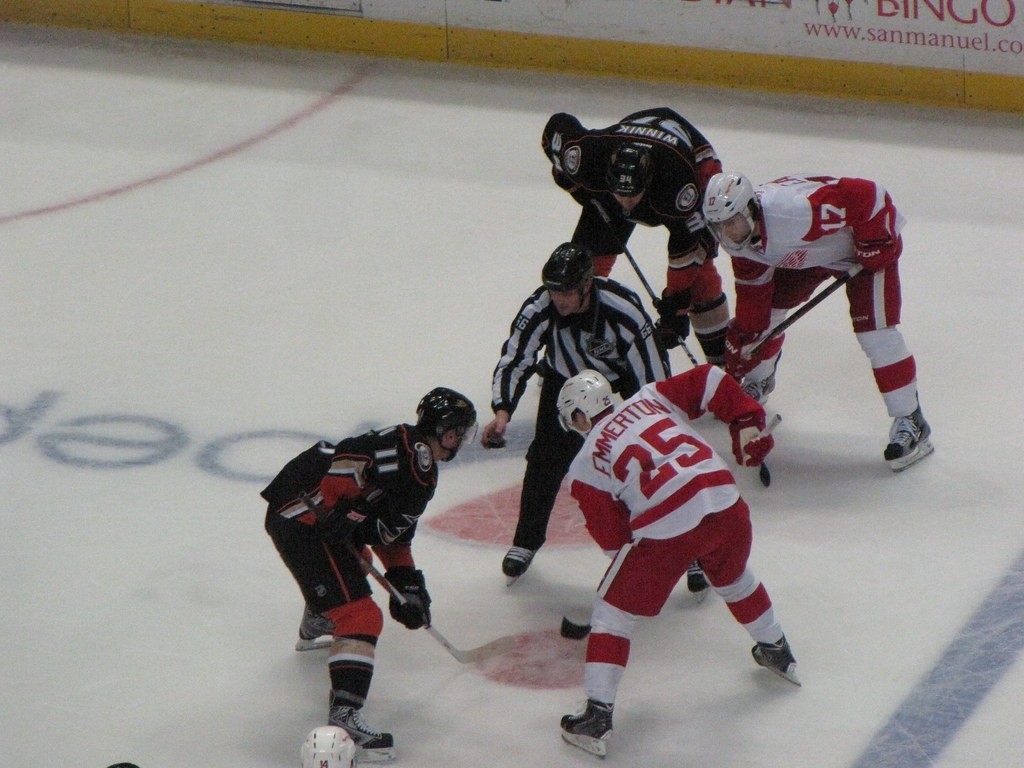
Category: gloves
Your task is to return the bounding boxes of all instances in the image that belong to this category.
[384,566,432,631]
[652,288,692,349]
[561,178,602,209]
[853,235,903,270]
[731,411,775,468]
[722,318,765,379]
[321,503,365,559]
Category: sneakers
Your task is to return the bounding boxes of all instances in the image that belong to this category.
[753,634,802,689]
[559,698,613,757]
[327,690,395,763]
[883,390,936,473]
[296,623,335,651]
[687,561,710,601]
[502,547,536,587]
[738,350,782,404]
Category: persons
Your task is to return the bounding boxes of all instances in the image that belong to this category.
[541,107,731,370]
[556,363,803,759]
[702,172,934,471]
[260,387,479,763]
[480,242,673,588]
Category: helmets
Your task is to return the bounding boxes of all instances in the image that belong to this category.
[557,370,612,428]
[702,173,757,221]
[606,141,651,196]
[416,389,478,440]
[541,241,592,292]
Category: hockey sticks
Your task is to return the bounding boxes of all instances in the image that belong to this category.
[559,413,783,641]
[725,263,863,372]
[590,195,699,367]
[295,490,517,665]
